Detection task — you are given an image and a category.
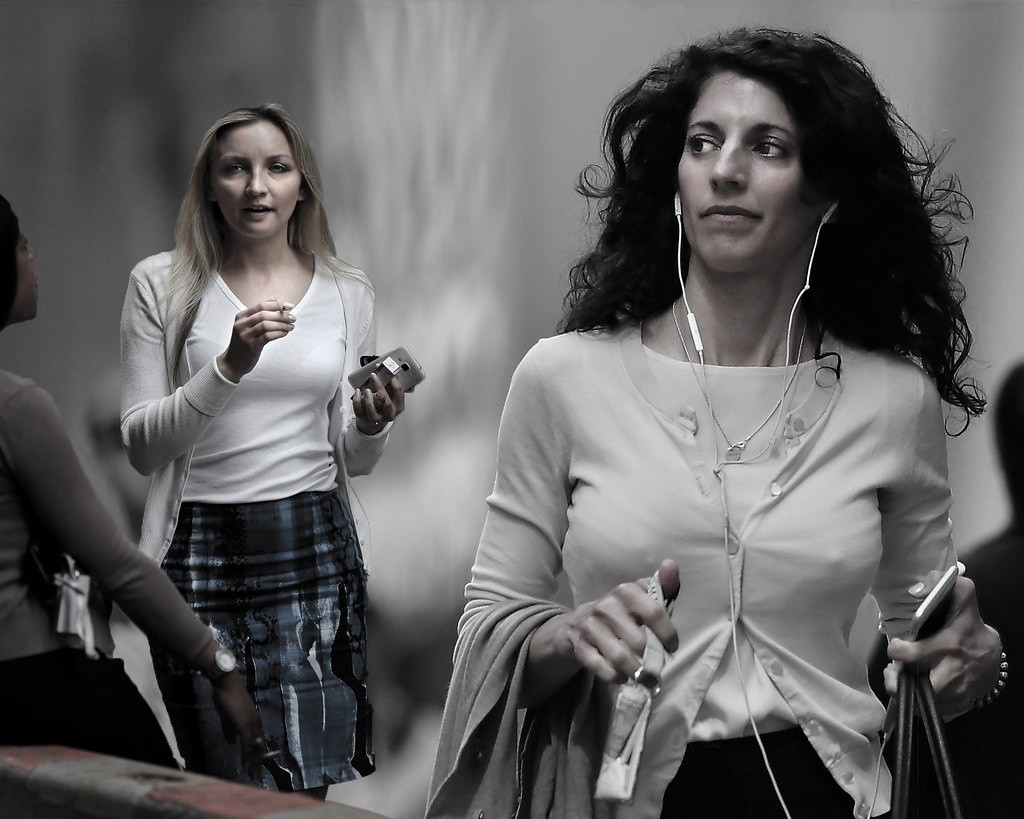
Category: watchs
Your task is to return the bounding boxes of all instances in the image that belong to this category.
[201,648,237,681]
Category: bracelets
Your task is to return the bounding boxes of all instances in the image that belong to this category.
[970,647,1007,710]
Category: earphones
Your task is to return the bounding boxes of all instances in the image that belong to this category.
[821,199,840,223]
[674,193,684,217]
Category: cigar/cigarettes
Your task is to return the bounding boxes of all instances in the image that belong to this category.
[278,304,287,314]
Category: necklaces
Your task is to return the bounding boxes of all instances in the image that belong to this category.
[671,299,809,463]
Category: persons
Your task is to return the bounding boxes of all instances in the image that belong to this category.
[0,195,264,774]
[120,100,415,802]
[421,28,1002,819]
[867,354,1024,819]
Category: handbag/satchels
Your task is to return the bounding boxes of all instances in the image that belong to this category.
[22,538,113,660]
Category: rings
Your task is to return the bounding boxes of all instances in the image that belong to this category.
[367,415,382,425]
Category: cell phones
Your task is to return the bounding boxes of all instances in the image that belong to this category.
[904,565,959,644]
[348,345,427,391]
